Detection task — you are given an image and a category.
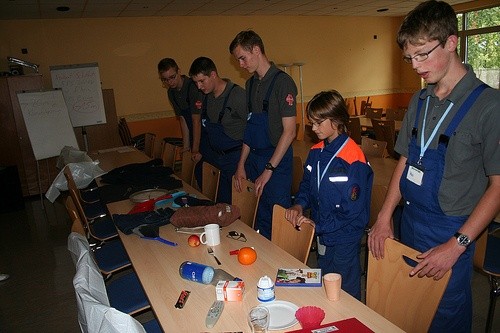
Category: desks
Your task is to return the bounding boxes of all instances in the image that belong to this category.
[346,113,402,131]
[88,146,407,333]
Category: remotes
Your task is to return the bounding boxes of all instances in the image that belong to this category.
[206,300,225,329]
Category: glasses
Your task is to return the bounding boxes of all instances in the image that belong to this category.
[308,119,329,127]
[226,231,248,242]
[402,43,443,64]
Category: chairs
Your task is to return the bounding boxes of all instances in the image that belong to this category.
[143,319,163,333]
[292,156,306,200]
[163,143,179,171]
[71,219,131,280]
[65,196,118,247]
[67,178,109,238]
[104,268,150,315]
[366,185,499,333]
[347,101,396,160]
[152,135,165,159]
[144,133,155,155]
[63,165,100,205]
[202,162,220,202]
[231,177,260,229]
[272,205,316,264]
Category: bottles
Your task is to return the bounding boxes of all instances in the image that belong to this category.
[179,261,243,286]
[257,276,275,302]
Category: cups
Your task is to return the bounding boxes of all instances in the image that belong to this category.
[200,224,220,246]
[249,307,269,333]
[323,273,342,302]
[295,306,325,329]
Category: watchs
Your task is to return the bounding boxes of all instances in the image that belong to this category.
[265,162,275,171]
[453,232,471,248]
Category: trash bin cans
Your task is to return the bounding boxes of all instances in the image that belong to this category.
[162,137,184,174]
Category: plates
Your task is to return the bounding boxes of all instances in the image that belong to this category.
[255,301,299,331]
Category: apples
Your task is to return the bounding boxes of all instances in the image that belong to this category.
[188,235,200,246]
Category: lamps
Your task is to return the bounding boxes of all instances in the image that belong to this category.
[277,62,291,72]
[294,60,305,132]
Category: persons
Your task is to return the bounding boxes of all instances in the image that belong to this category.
[368,0,500,333]
[284,89,373,303]
[157,31,296,241]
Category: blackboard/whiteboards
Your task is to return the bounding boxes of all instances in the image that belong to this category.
[19,92,80,161]
[51,63,108,128]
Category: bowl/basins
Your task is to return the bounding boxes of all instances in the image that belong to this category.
[129,188,169,203]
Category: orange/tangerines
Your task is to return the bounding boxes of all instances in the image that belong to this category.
[238,247,257,265]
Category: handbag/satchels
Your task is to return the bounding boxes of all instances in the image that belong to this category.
[101,158,164,186]
